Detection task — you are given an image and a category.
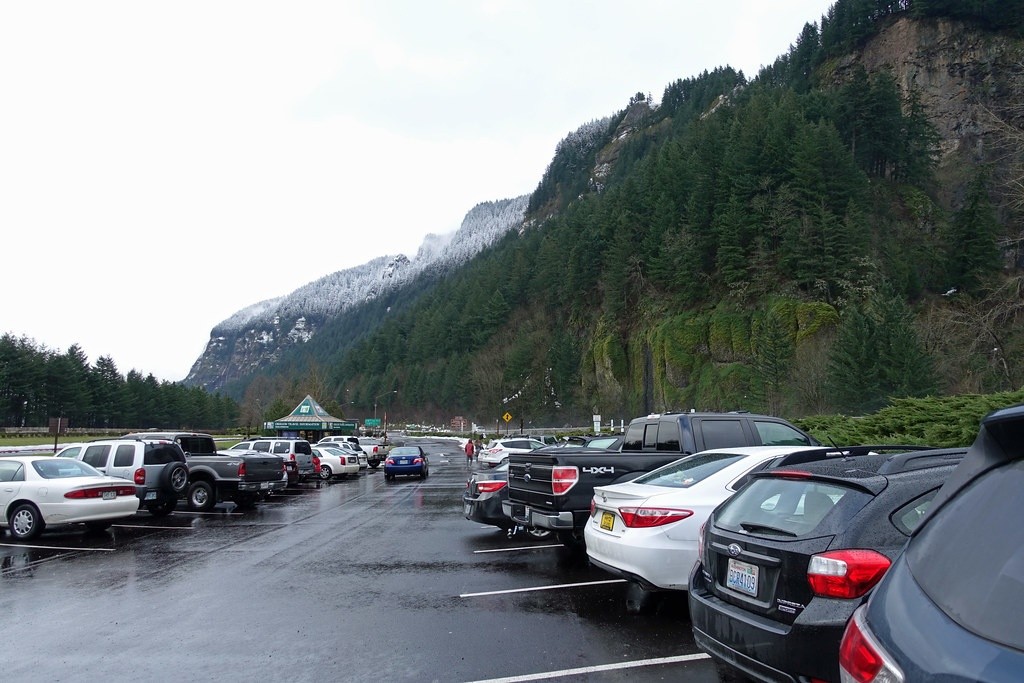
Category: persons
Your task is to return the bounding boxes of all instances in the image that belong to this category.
[465,436,484,467]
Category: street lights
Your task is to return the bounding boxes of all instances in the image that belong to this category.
[375,390,397,434]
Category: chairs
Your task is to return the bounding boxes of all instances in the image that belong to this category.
[803,491,834,522]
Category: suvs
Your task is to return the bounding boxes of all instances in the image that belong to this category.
[688,444,974,683]
[228,435,313,487]
[53,437,189,518]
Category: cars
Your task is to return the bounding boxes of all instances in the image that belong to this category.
[584,445,880,593]
[462,437,624,535]
[217,449,302,502]
[309,443,369,481]
[384,446,429,482]
[838,402,1023,683]
[0,456,140,542]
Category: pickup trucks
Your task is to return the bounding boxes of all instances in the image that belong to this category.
[121,431,286,512]
[309,435,389,469]
[501,411,830,556]
